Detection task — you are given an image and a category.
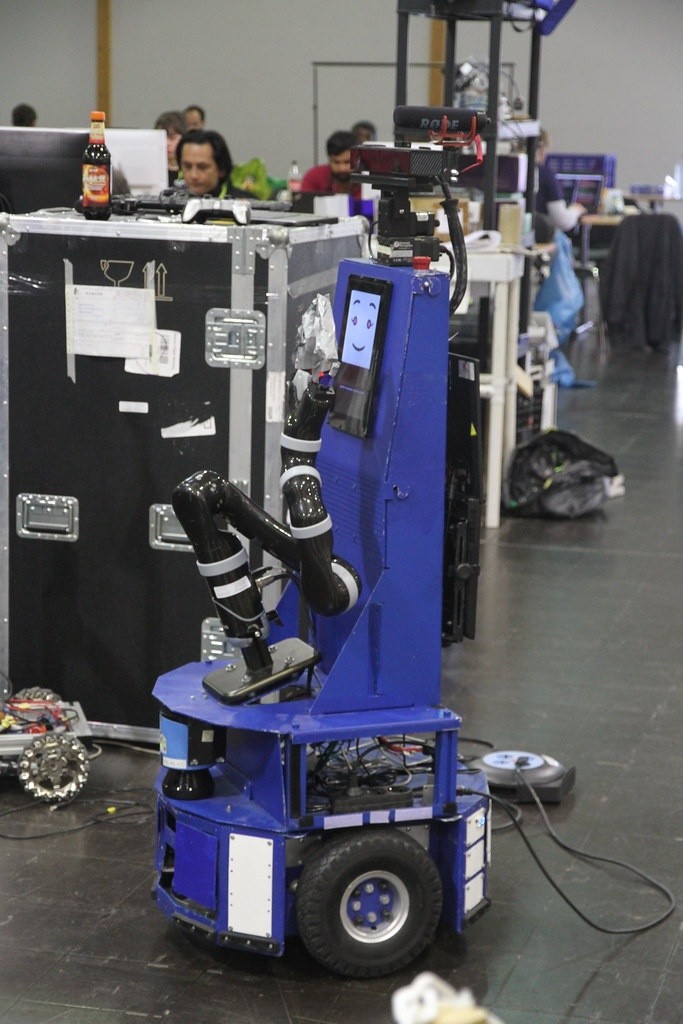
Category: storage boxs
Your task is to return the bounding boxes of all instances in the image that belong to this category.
[0,206,373,756]
[546,152,617,193]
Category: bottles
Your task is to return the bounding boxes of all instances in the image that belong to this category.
[82,111,112,221]
[287,160,304,202]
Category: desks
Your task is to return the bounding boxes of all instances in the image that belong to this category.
[571,216,623,258]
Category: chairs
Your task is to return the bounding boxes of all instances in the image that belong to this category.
[600,213,683,353]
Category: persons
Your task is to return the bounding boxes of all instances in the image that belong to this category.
[12,103,36,126]
[154,106,258,200]
[303,122,375,198]
[512,127,589,386]
